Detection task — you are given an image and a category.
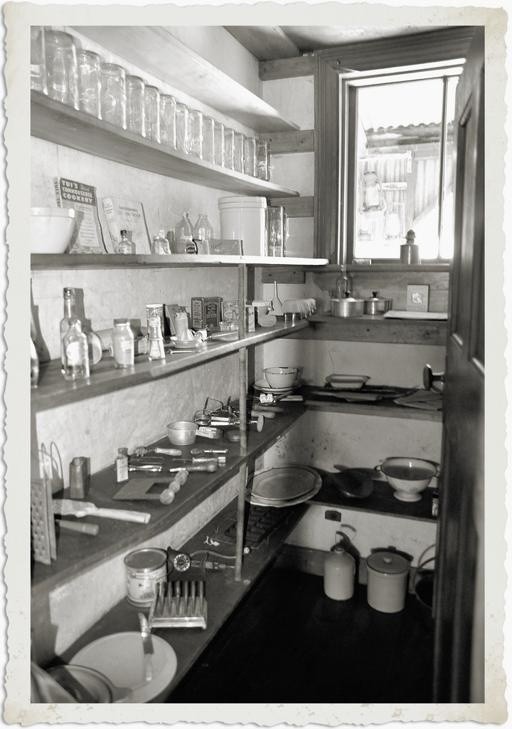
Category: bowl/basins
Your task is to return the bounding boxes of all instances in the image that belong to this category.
[31,207,76,254]
[166,421,214,446]
[262,367,297,389]
[379,457,437,503]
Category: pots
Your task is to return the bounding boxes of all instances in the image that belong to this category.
[47,664,133,703]
[328,291,364,318]
[364,291,392,315]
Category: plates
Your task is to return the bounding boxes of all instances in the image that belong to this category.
[245,464,323,507]
[251,380,302,394]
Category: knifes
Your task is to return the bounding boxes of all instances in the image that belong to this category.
[138,612,154,682]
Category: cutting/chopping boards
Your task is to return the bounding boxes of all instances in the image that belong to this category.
[70,631,178,703]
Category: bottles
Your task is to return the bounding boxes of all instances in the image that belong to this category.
[114,448,130,483]
[118,230,132,254]
[175,313,189,343]
[323,545,356,601]
[59,288,166,381]
[366,551,409,615]
[152,212,213,255]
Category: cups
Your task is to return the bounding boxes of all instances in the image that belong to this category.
[31,26,270,181]
